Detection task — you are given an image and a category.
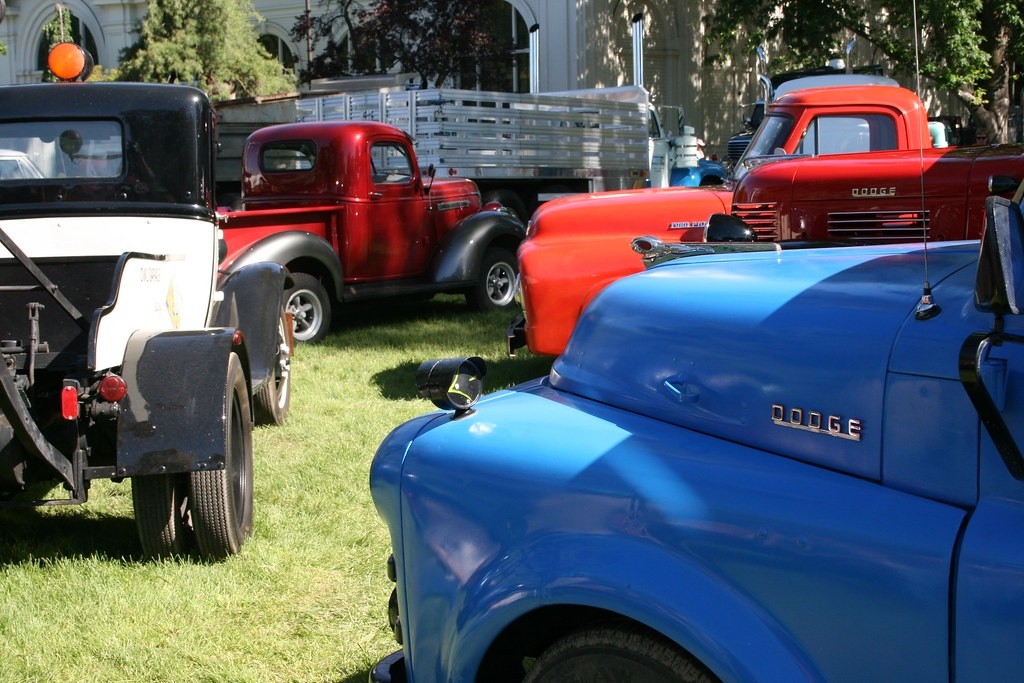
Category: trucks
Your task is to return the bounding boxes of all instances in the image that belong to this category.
[1,37,295,563]
[368,173,1023,683]
[500,64,1024,356]
[214,120,478,340]
[276,79,735,256]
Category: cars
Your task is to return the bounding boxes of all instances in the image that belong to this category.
[0,147,46,179]
